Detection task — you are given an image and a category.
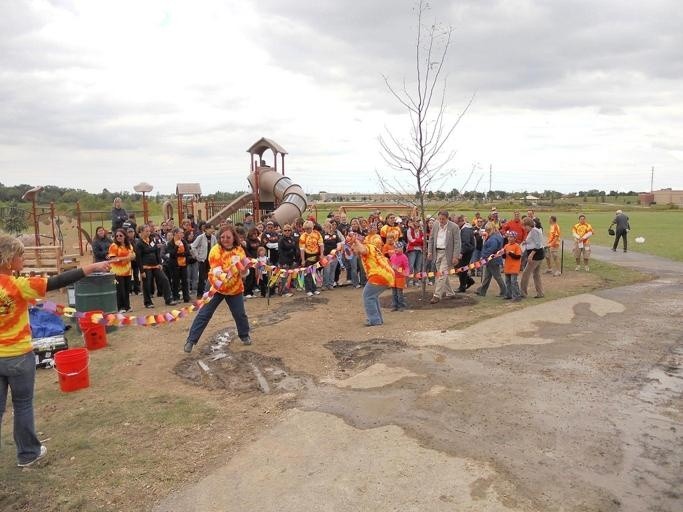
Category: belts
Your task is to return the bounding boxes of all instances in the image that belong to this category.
[436,247,445,251]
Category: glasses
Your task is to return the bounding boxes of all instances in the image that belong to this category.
[283,229,291,232]
[115,234,125,238]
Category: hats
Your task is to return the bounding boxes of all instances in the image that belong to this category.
[500,218,507,224]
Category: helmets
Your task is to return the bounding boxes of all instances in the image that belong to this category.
[504,229,518,237]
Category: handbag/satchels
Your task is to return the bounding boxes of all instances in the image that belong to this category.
[608,228,615,235]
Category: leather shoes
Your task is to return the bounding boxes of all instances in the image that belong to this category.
[428,295,439,305]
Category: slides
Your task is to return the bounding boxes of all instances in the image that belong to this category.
[258,169,307,226]
[206,192,252,226]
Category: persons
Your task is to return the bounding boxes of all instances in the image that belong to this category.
[110,197,128,235]
[572,214,593,271]
[367,211,479,301]
[243,213,367,297]
[224,217,247,248]
[347,234,393,328]
[545,215,561,276]
[0,233,121,467]
[471,211,544,301]
[93,211,218,313]
[608,210,630,253]
[182,225,251,352]
[388,241,409,310]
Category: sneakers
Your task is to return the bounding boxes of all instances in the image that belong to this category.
[167,300,178,305]
[465,277,474,290]
[241,336,252,345]
[118,307,133,313]
[453,285,465,293]
[244,281,369,299]
[184,340,193,353]
[474,290,544,302]
[14,443,47,467]
[407,281,434,289]
[144,304,155,308]
[541,266,590,277]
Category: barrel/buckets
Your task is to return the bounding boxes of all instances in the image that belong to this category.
[53,348,91,391]
[78,311,107,350]
[74,272,118,332]
